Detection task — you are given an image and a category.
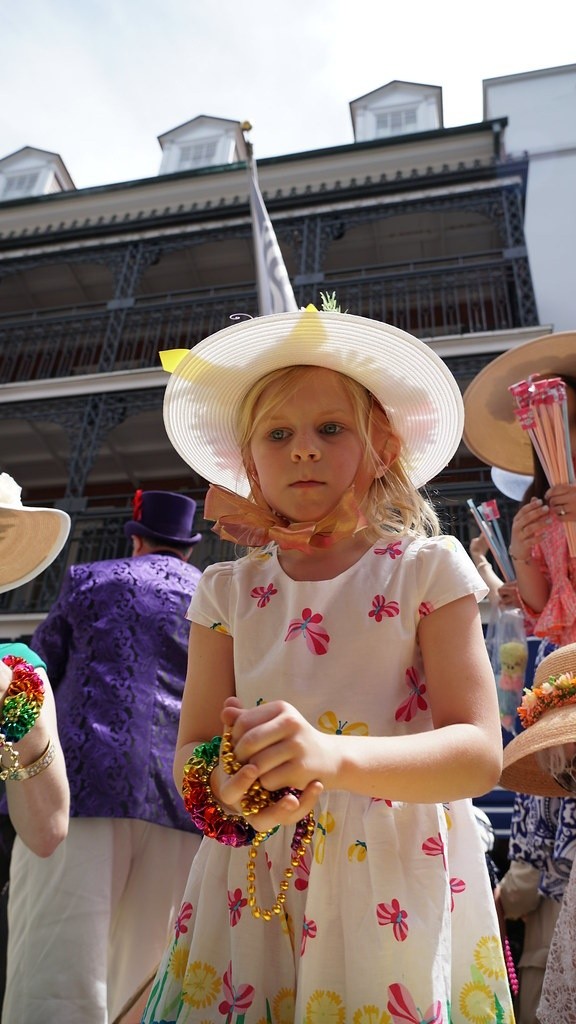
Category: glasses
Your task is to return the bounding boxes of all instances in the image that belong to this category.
[553,754,576,792]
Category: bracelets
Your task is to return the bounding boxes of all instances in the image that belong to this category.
[182,738,281,846]
[0,655,45,742]
[0,735,56,781]
[202,758,246,823]
[508,549,531,563]
[477,562,492,570]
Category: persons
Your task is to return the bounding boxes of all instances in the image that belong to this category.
[3,491,204,1023]
[0,472,72,857]
[464,332,576,1024]
[142,303,517,1024]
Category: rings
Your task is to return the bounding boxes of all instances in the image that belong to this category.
[560,505,565,515]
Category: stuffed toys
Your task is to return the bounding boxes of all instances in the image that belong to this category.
[496,642,525,728]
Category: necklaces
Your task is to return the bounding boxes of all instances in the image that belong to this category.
[222,733,314,920]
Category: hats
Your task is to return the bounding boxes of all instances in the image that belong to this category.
[463,331,576,476]
[496,642,576,798]
[159,312,465,553]
[0,472,71,594]
[124,491,202,549]
